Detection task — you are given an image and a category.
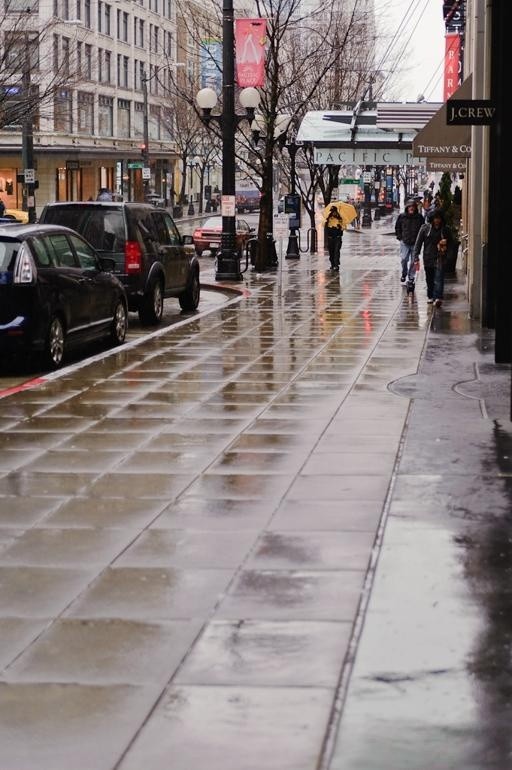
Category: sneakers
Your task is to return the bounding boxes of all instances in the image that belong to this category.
[330,266,339,271]
[427,298,442,309]
[401,276,414,283]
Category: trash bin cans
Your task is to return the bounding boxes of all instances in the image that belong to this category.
[173,206,183,219]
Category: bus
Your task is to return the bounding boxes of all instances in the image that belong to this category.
[235,158,280,213]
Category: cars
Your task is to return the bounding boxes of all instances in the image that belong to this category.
[0,202,200,370]
[277,190,324,214]
[192,217,256,255]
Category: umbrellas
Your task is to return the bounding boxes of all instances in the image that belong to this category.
[321,201,357,225]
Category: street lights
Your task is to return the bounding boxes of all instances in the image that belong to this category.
[22,19,85,223]
[141,63,185,202]
[196,85,260,281]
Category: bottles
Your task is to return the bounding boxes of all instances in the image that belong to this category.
[414,258,420,273]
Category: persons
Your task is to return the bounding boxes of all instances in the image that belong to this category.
[324,206,343,271]
[395,181,447,308]
[345,194,361,230]
[97,188,113,203]
[213,185,220,193]
[0,198,5,218]
